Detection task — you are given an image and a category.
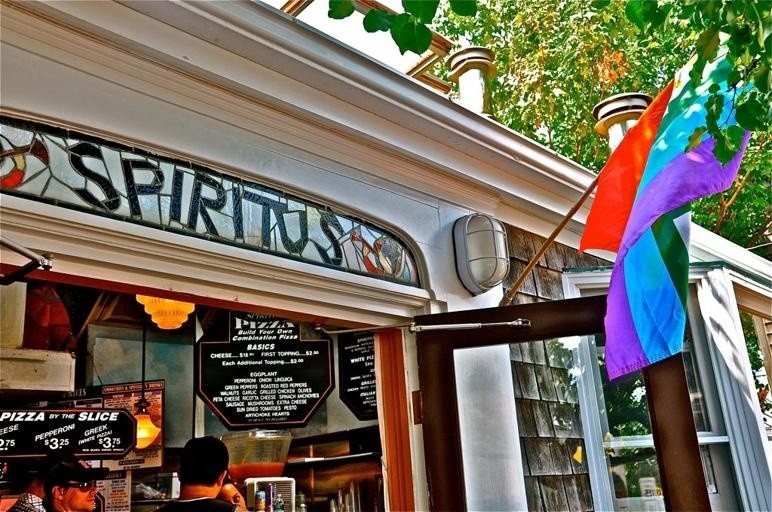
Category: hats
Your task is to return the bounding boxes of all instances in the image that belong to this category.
[44,458,109,483]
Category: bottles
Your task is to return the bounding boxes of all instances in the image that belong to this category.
[273,492,285,512]
[298,503,308,512]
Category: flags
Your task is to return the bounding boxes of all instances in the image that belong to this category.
[575,39,758,384]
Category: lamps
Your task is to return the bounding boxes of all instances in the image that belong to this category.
[134,294,196,331]
[133,313,160,451]
[453,212,510,298]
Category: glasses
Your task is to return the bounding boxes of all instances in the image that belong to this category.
[65,481,96,492]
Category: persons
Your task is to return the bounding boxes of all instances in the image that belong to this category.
[218,481,248,511]
[43,454,100,512]
[153,436,242,511]
[7,453,77,512]
